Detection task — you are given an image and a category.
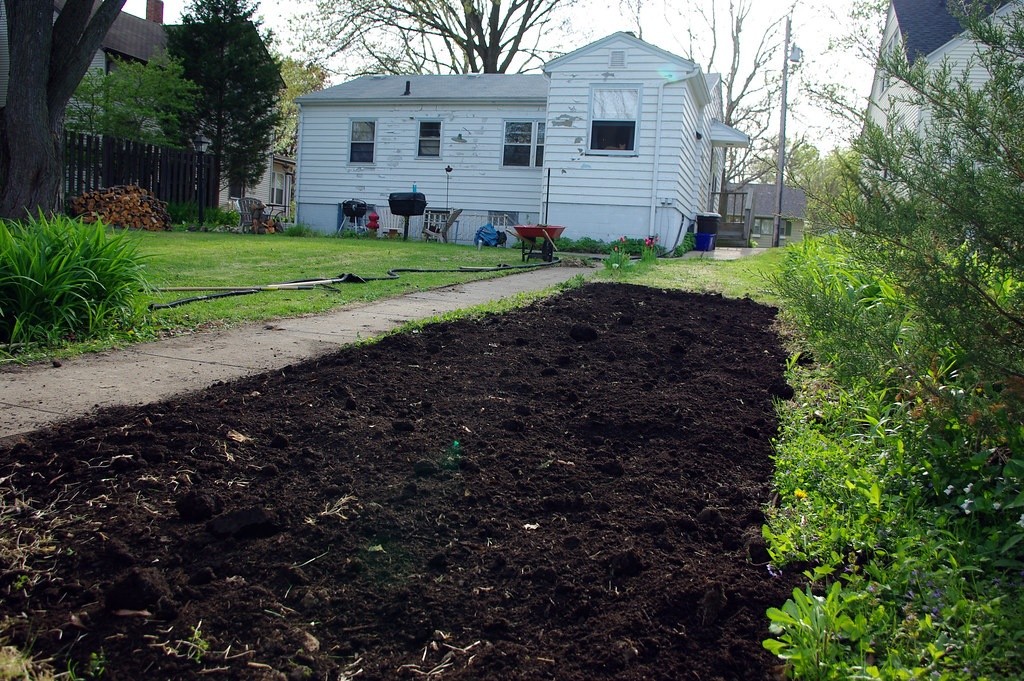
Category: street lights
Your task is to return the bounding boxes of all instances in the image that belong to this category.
[192,130,211,226]
[773,44,802,248]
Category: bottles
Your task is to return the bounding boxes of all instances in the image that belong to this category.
[413,181,417,192]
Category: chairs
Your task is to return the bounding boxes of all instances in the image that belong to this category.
[238,197,283,235]
[422,209,463,244]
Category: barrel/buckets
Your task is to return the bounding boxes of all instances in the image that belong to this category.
[694,230,717,251]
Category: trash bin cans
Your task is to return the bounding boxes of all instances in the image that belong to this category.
[696,212,722,251]
